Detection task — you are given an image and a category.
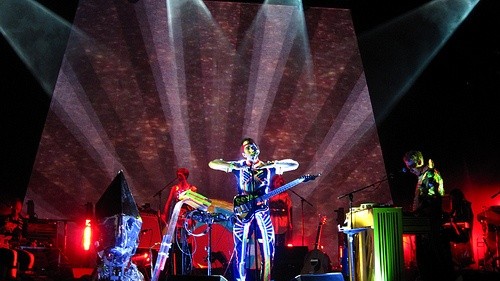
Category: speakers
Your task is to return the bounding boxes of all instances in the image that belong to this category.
[272,246,308,281]
[300,249,332,275]
[165,275,228,281]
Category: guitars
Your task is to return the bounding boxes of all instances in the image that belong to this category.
[232,173,321,224]
[301,215,334,275]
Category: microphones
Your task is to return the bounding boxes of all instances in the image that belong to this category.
[391,168,407,179]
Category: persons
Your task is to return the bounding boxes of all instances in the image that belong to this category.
[208,138,300,280]
[5,200,27,244]
[268,173,292,247]
[442,189,474,256]
[403,150,445,281]
[161,168,196,276]
